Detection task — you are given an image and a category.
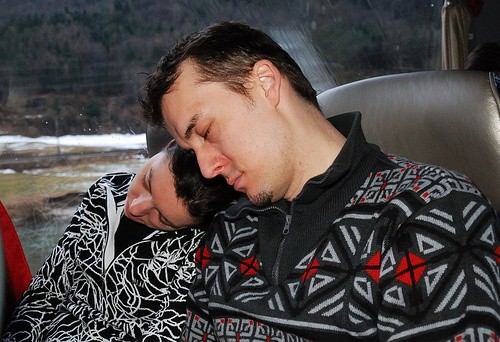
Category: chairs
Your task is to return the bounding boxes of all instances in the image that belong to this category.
[317,68,500,223]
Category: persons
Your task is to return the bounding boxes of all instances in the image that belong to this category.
[136,21,500,342]
[0,137,240,342]
